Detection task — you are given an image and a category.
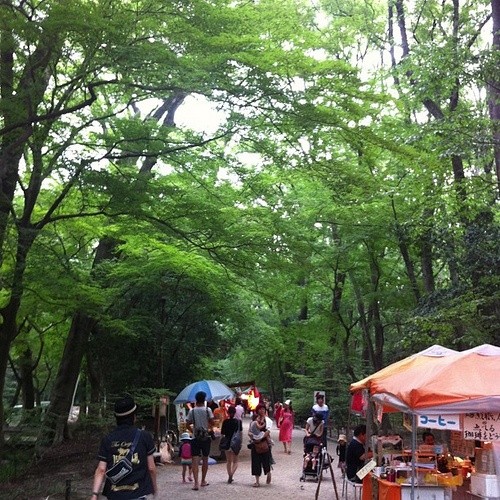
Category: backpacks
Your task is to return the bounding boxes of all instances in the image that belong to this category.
[252,442,269,453]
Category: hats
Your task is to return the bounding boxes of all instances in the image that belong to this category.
[337,434,348,443]
[114,398,138,420]
[283,399,293,406]
[180,432,192,441]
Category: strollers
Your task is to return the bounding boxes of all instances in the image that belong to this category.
[300,434,327,482]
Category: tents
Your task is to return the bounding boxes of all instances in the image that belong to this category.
[350,344,500,500]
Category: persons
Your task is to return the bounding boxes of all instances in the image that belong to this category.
[422,432,435,445]
[248,404,276,487]
[345,424,373,483]
[311,392,329,451]
[179,433,193,484]
[184,391,215,490]
[274,401,283,428]
[251,415,275,446]
[263,397,273,418]
[91,398,158,500]
[336,434,348,479]
[279,400,295,455]
[303,413,326,454]
[303,445,320,470]
[219,405,243,483]
[185,392,246,425]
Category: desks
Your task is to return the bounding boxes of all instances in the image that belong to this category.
[362,471,445,500]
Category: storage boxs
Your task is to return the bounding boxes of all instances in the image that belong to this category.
[471,472,500,498]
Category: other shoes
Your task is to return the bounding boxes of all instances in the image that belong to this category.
[251,482,260,486]
[201,482,209,485]
[227,478,234,483]
[266,474,271,484]
[182,476,192,483]
[282,449,291,454]
[192,487,199,491]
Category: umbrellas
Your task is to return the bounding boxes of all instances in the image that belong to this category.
[173,379,234,403]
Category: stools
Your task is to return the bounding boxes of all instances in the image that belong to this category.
[342,478,363,500]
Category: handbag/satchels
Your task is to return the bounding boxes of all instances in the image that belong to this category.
[230,419,243,456]
[105,429,143,484]
[194,428,210,444]
